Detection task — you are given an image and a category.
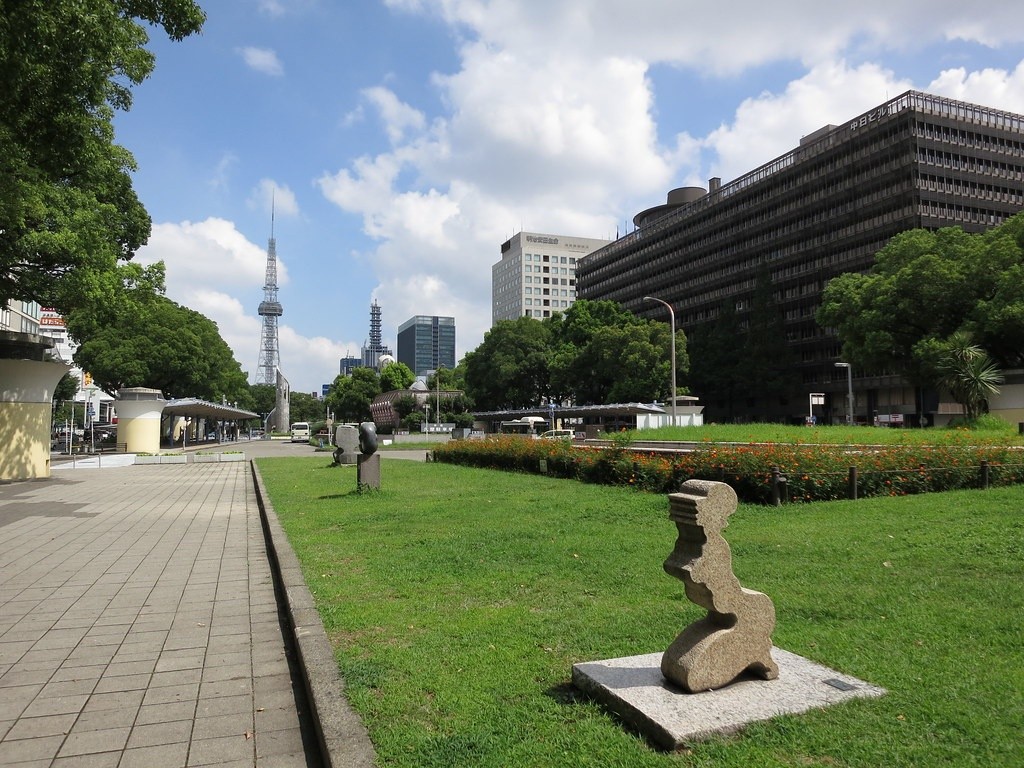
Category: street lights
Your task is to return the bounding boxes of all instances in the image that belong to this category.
[435,363,445,423]
[644,296,676,427]
[834,362,854,426]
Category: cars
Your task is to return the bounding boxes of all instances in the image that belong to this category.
[59,431,79,444]
[83,431,92,440]
[209,433,214,437]
[51,432,58,450]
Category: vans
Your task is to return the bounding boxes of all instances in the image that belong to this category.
[537,429,575,439]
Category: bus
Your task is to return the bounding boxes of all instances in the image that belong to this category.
[498,416,549,439]
[290,422,311,443]
